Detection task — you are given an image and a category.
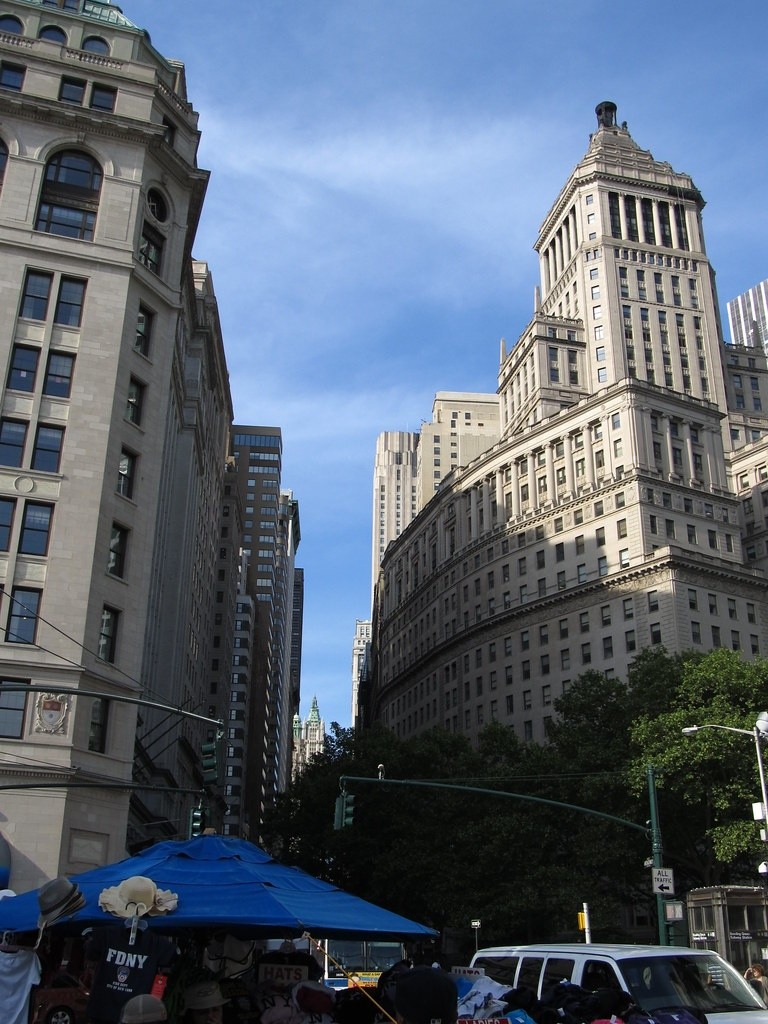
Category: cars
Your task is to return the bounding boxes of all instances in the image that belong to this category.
[28,964,90,1024]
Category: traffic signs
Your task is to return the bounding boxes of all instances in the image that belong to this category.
[471,920,481,929]
[652,867,674,895]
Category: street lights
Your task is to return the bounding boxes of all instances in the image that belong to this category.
[682,724,767,822]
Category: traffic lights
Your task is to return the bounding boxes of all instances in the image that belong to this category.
[578,913,585,929]
[202,732,226,786]
[343,795,356,830]
[751,802,765,821]
[664,901,685,922]
[190,808,203,839]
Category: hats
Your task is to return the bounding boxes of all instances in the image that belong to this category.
[187,981,231,1009]
[37,876,86,928]
[122,995,167,1024]
[98,876,178,920]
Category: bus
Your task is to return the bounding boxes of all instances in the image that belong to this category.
[267,936,406,991]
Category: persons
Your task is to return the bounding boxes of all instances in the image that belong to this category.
[744,964,768,1006]
[179,982,231,1024]
[122,994,169,1024]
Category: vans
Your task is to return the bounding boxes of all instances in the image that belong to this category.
[472,944,767,1024]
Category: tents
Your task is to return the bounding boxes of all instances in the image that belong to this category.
[0,828,442,936]
